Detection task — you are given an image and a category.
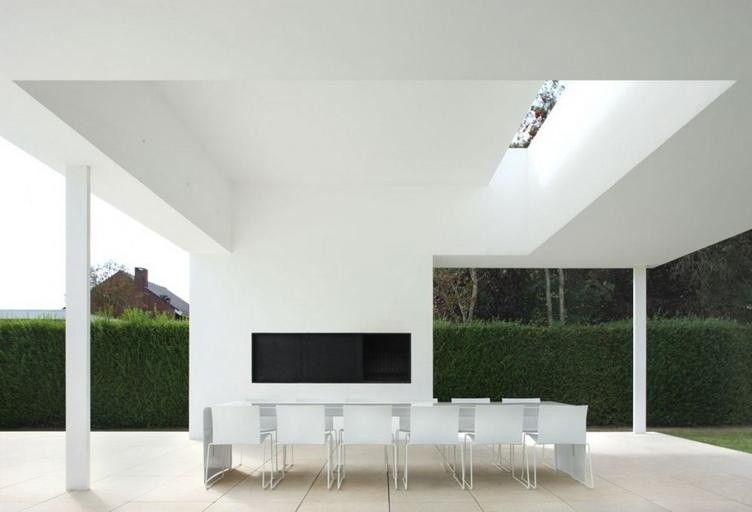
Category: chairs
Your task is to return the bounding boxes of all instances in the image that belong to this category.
[445,398,505,473]
[337,405,398,490]
[500,397,558,473]
[464,404,531,490]
[398,398,451,472]
[269,405,334,490]
[521,403,594,489]
[402,405,465,490]
[205,405,274,491]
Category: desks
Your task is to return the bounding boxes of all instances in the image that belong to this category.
[202,403,587,491]
